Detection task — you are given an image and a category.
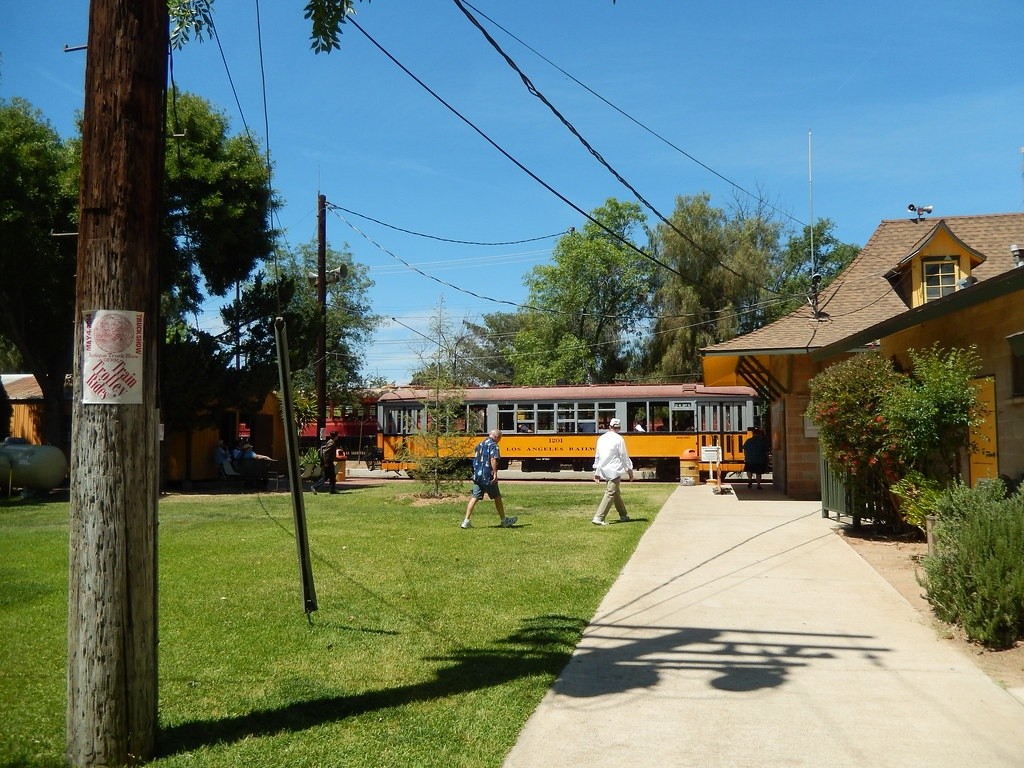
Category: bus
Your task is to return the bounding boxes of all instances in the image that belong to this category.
[372,385,761,480]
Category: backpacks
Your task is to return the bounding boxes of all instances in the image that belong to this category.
[318,441,333,468]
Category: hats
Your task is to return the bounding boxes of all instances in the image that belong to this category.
[242,444,253,449]
[637,418,643,421]
[216,440,223,446]
[234,437,244,444]
[610,418,621,428]
[752,427,761,433]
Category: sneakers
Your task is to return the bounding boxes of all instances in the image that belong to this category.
[329,490,338,494]
[501,516,518,528]
[311,486,317,495]
[620,517,630,522]
[592,517,609,525]
[461,520,471,529]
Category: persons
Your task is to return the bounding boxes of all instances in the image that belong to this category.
[592,418,633,525]
[311,431,338,495]
[461,429,518,529]
[518,424,533,432]
[743,427,769,489]
[633,420,647,432]
[216,438,270,493]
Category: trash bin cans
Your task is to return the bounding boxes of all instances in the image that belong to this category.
[334,449,347,482]
[679,449,699,486]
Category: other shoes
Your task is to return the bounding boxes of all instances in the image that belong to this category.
[757,486,763,490]
[748,481,752,489]
